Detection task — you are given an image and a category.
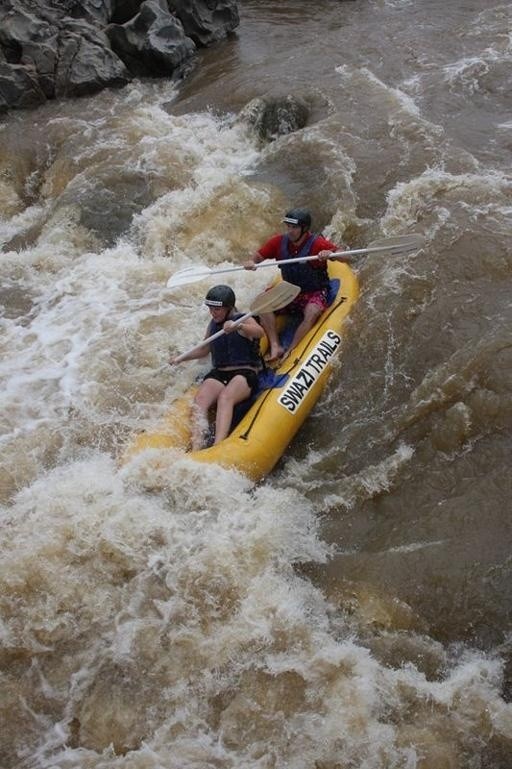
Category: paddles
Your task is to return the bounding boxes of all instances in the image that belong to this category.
[159,280,301,371]
[167,233,426,287]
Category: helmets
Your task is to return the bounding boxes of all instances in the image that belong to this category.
[280,208,312,230]
[203,285,236,310]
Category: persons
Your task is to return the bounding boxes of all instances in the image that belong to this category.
[169,285,264,455]
[244,208,349,362]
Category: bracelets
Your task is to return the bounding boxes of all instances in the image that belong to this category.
[238,323,243,330]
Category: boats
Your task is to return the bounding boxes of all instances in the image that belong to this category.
[121,259,360,481]
